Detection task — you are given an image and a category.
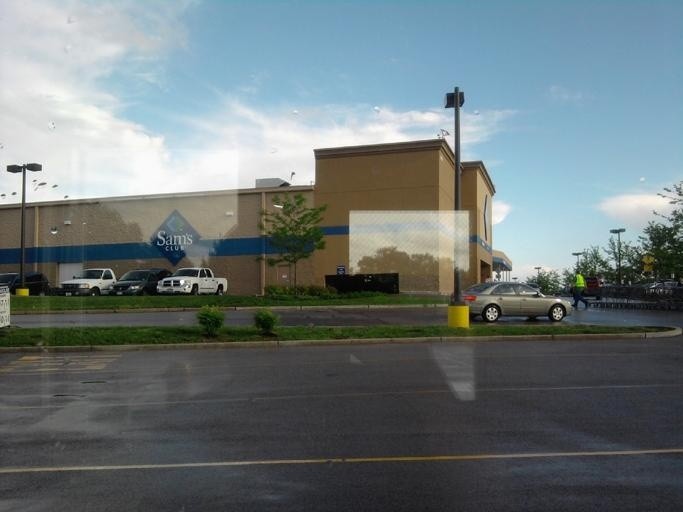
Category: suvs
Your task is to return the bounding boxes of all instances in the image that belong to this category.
[570,275,603,301]
[106,268,173,298]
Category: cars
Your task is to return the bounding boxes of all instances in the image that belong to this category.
[521,281,543,295]
[628,277,677,300]
[459,279,573,323]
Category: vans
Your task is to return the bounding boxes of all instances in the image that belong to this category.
[0,270,52,297]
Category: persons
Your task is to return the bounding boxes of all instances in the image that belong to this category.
[570,277,577,299]
[571,270,590,309]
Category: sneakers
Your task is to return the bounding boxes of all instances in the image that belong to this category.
[572,302,589,309]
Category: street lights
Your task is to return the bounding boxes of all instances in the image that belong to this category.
[608,226,625,286]
[571,250,582,273]
[533,266,541,278]
[442,83,471,334]
[5,161,44,297]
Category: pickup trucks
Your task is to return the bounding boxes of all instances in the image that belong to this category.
[149,265,229,297]
[58,266,120,297]
